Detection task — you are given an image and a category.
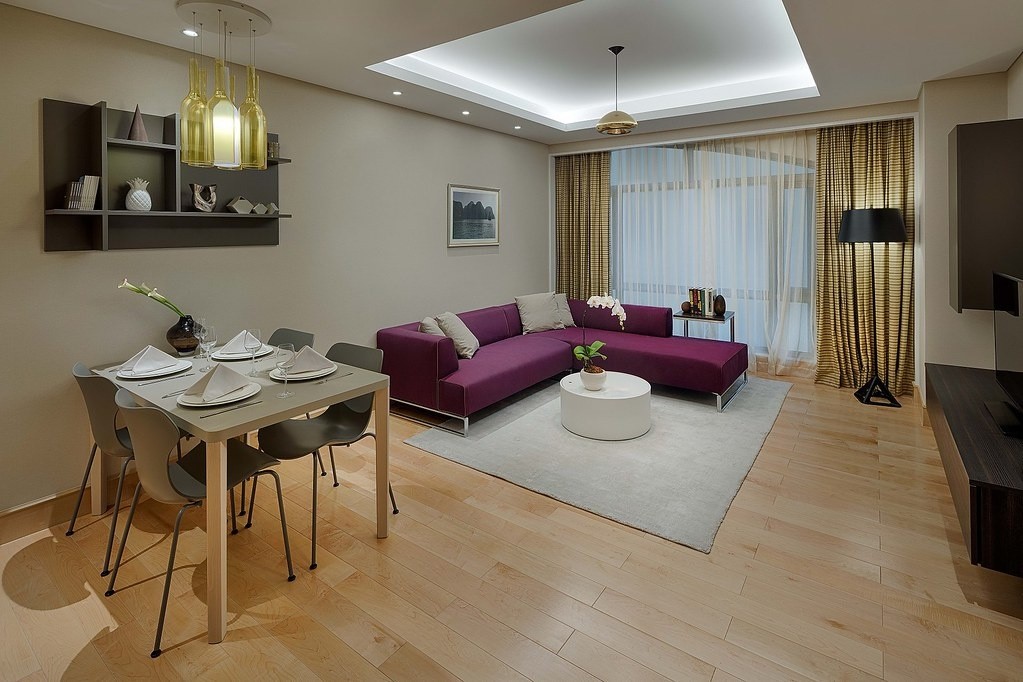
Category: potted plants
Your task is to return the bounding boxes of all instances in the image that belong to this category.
[575,340,609,391]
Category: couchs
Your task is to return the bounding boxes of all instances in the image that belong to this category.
[377,296,749,438]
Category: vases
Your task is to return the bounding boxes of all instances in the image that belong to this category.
[165,314,211,358]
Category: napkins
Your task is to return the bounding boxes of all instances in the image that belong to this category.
[219,331,268,357]
[279,345,335,373]
[182,364,251,402]
[116,344,180,377]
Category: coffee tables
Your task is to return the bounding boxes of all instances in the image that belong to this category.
[559,370,651,440]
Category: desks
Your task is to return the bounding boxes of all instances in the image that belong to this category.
[673,309,736,342]
[86,348,391,642]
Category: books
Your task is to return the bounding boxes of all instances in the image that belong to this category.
[689,287,717,316]
[65,175,99,211]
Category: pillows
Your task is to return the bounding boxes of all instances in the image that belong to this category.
[553,294,576,327]
[514,290,565,334]
[419,316,446,336]
[437,311,480,359]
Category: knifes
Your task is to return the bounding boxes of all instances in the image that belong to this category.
[314,372,354,384]
[200,400,264,419]
[138,373,195,386]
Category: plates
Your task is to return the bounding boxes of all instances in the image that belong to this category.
[177,380,261,408]
[269,362,338,380]
[210,345,272,360]
[117,359,193,379]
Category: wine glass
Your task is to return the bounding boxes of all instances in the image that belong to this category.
[243,329,262,378]
[199,326,217,373]
[275,343,295,399]
[192,312,210,358]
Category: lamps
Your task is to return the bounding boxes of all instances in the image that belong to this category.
[177,0,272,168]
[838,209,910,408]
[595,45,639,134]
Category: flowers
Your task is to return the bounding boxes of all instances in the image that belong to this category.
[118,275,193,322]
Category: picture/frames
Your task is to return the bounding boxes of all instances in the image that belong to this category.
[447,184,500,249]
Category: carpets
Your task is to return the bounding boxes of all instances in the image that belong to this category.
[404,376,794,554]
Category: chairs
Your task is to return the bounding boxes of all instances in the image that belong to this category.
[65,360,185,578]
[106,389,295,660]
[246,340,400,569]
[229,329,328,518]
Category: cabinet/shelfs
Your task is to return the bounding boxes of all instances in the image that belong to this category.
[40,98,295,253]
[949,119,1023,313]
[924,362,1023,579]
